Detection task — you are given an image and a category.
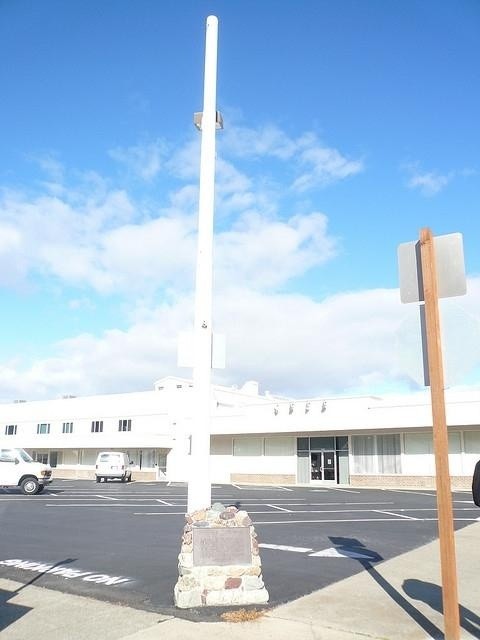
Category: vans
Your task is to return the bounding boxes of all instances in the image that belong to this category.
[94,451,133,483]
[0,445,53,494]
